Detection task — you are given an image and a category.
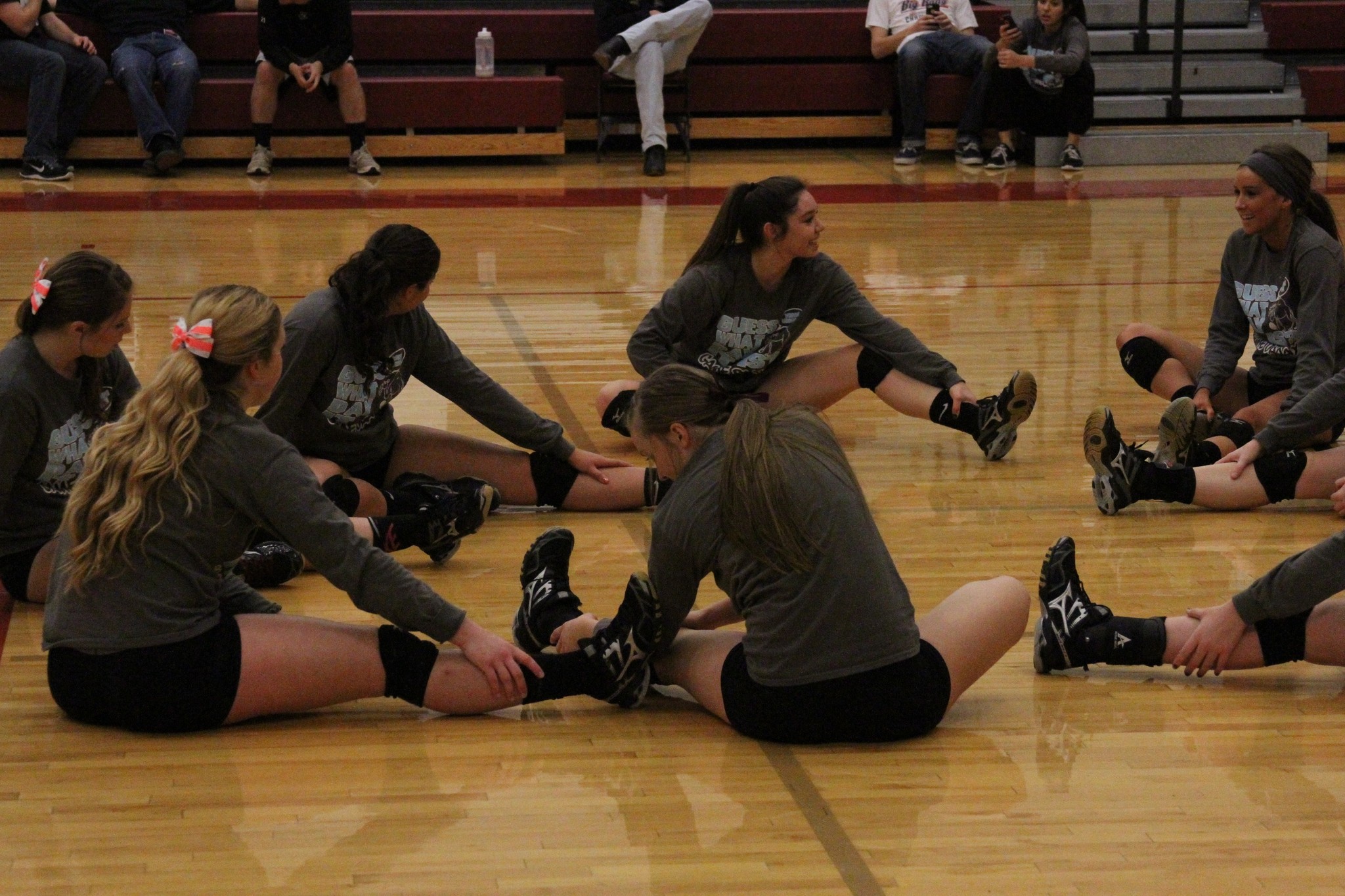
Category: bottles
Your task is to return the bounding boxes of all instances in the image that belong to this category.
[476,27,495,78]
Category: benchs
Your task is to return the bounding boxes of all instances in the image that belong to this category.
[1262,0,1345,117]
[0,0,1013,158]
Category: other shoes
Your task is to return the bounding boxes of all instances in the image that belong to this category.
[142,150,172,176]
[644,149,665,175]
[592,42,619,68]
[158,140,186,170]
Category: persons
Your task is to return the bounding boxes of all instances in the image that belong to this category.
[250,223,675,521]
[984,0,1095,172]
[513,363,1035,746]
[1116,141,1345,471]
[47,0,260,171]
[865,0,995,165]
[40,286,660,736]
[1032,361,1345,677]
[0,249,493,611]
[596,176,1038,460]
[0,0,97,181]
[593,0,714,176]
[247,0,383,176]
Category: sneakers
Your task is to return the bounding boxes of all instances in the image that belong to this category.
[356,174,381,190]
[955,144,983,165]
[1083,405,1154,515]
[1060,144,1083,171]
[1151,396,1197,469]
[1192,412,1228,444]
[232,540,305,587]
[512,527,583,654]
[984,168,1016,181]
[642,176,670,199]
[975,368,1037,461]
[247,175,271,193]
[1061,170,1084,184]
[893,147,924,164]
[349,141,381,175]
[957,164,983,175]
[246,143,271,175]
[1033,535,1113,673]
[578,571,662,708]
[21,179,73,198]
[984,142,1017,168]
[20,161,74,181]
[414,484,493,565]
[393,471,501,515]
[57,157,74,170]
[894,164,924,175]
